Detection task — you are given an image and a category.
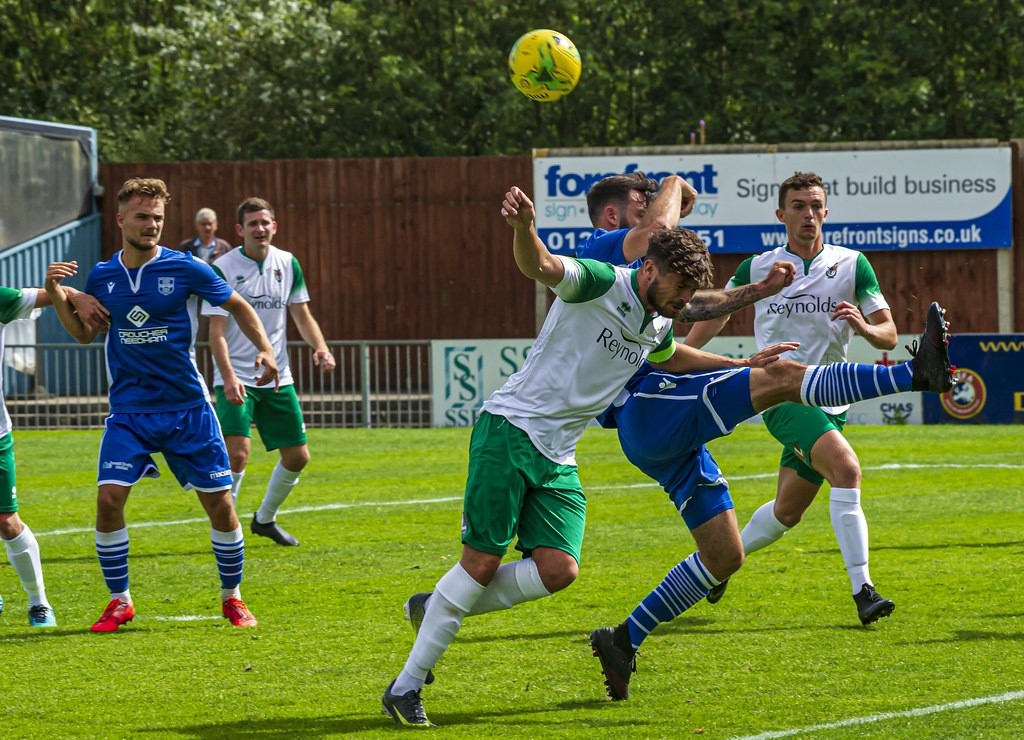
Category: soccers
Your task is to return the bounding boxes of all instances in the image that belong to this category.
[508,29,582,102]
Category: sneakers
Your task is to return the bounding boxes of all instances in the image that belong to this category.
[90,597,136,632]
[706,576,732,603]
[28,603,57,627]
[852,583,896,625]
[402,592,436,685]
[904,301,957,394]
[250,512,300,546]
[379,678,432,729]
[221,596,258,627]
[588,627,642,701]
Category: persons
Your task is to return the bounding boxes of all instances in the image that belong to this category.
[574,174,955,702]
[178,206,233,392]
[684,170,898,624]
[198,198,335,548]
[45,177,280,632]
[382,185,796,729]
[0,285,111,627]
